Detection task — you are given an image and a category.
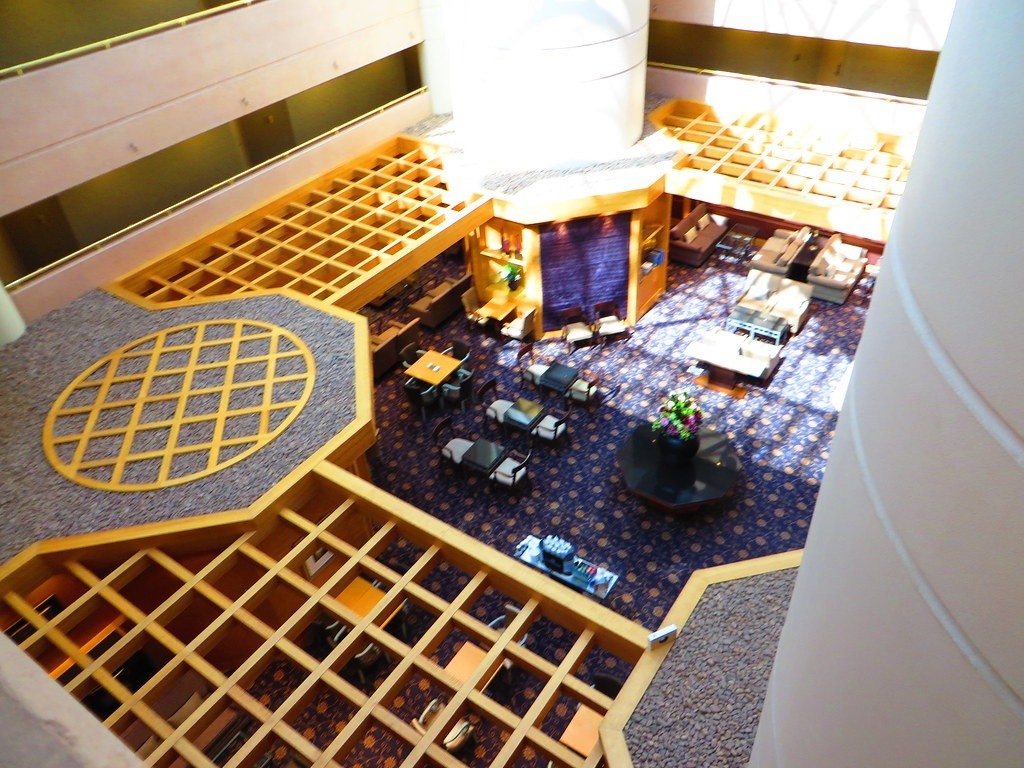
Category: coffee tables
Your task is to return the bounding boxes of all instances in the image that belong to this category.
[716,222,760,264]
[211,731,274,768]
[726,305,790,346]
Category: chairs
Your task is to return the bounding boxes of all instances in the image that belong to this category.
[324,559,624,768]
[489,448,531,497]
[476,377,514,442]
[429,416,475,478]
[460,287,494,340]
[530,404,573,452]
[500,307,538,349]
[517,299,631,418]
[399,343,478,425]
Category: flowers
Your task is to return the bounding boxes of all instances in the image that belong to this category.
[651,390,706,441]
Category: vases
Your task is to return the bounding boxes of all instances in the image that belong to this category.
[658,430,700,460]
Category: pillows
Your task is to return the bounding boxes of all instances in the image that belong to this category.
[772,244,788,264]
[134,735,176,768]
[697,212,711,231]
[824,262,836,279]
[783,230,799,245]
[684,226,699,244]
[833,251,847,264]
[168,691,203,728]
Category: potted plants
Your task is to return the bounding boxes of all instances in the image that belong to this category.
[500,263,523,291]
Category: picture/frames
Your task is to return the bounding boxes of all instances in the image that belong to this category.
[5,595,76,660]
[291,512,337,583]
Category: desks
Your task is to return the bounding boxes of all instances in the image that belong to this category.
[504,396,547,443]
[461,435,506,487]
[403,350,462,415]
[445,640,505,693]
[357,306,384,331]
[336,576,373,609]
[384,270,438,309]
[352,585,404,631]
[558,702,604,757]
[539,361,580,410]
[477,296,517,342]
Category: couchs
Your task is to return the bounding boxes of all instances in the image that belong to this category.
[749,226,812,278]
[371,317,421,383]
[736,269,815,334]
[410,273,473,332]
[807,234,869,305]
[669,201,733,269]
[111,662,254,768]
[683,326,785,383]
